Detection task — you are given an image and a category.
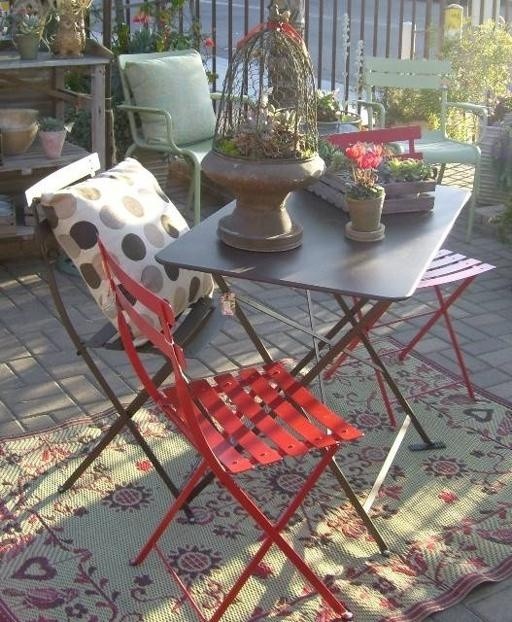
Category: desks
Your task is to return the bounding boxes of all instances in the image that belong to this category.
[154,184,472,559]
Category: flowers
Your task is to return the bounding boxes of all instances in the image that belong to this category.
[350,141,383,194]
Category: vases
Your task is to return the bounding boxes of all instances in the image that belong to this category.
[343,196,387,245]
[0,106,39,157]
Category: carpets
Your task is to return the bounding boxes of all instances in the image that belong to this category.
[0,336,510,622]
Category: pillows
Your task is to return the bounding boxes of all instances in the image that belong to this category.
[43,154,213,349]
[127,53,217,146]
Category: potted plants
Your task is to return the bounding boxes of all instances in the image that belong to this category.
[50,0,93,58]
[38,118,67,156]
[11,15,46,59]
[315,90,361,132]
[500,96,512,126]
[197,7,326,253]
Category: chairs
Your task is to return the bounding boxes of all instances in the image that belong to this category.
[92,230,363,621]
[27,152,296,522]
[353,54,489,244]
[324,126,497,428]
[116,46,256,228]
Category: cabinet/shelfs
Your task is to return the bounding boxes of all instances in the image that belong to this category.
[0,34,114,262]
